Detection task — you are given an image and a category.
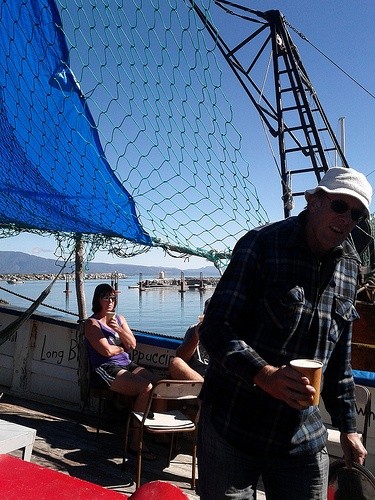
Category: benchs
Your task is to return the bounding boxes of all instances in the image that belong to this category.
[0,454,130,500]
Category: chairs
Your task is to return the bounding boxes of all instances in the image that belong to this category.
[123,380,203,488]
[78,323,133,435]
[327,384,372,468]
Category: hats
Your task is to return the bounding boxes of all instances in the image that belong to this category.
[305,167,375,221]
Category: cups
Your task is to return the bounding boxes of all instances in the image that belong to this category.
[289,359,324,406]
[199,315,204,321]
[105,312,117,324]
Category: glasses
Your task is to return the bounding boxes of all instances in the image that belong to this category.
[102,295,116,302]
[310,193,363,221]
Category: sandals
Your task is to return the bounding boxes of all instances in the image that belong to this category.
[129,438,159,460]
[160,438,183,454]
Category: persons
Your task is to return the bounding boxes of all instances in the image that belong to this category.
[174,167,373,500]
[83,283,174,461]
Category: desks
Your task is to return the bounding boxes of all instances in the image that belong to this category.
[0,418,37,463]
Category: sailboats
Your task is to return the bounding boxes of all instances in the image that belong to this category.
[0,1,375,500]
